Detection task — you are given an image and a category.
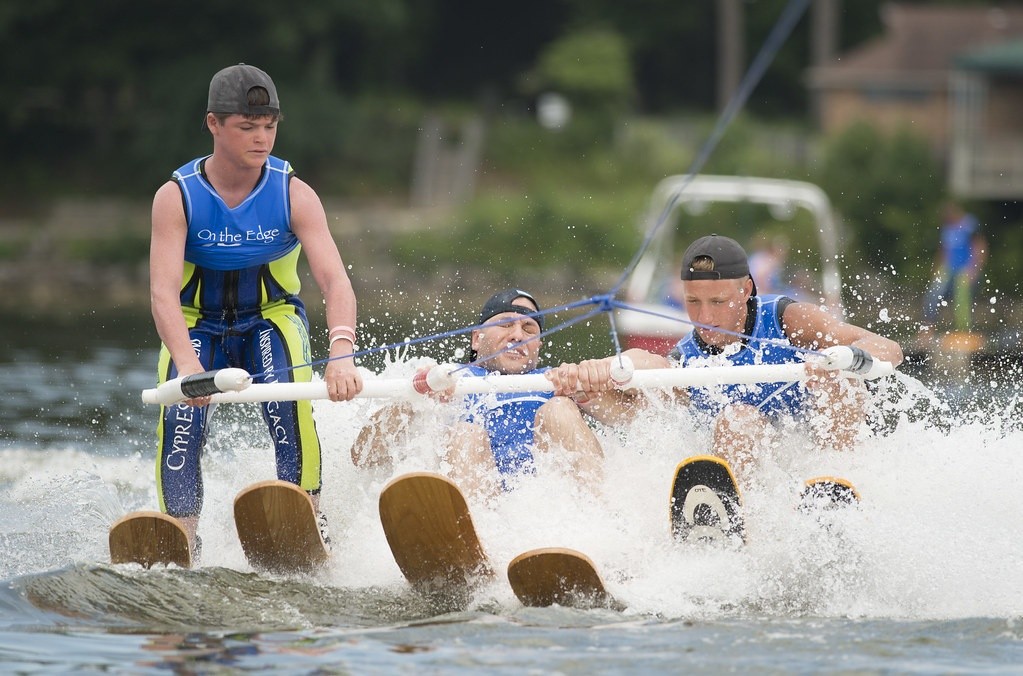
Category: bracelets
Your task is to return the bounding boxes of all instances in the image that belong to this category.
[328,326,357,341]
[329,335,354,349]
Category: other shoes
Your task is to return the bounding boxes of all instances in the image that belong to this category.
[314,512,334,552]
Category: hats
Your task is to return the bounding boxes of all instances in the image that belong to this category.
[680,234,757,298]
[200,62,280,133]
[469,288,544,364]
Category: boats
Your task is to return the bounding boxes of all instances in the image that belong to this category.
[610,176,849,364]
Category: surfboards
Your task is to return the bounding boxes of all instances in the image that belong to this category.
[378,470,628,614]
[108,478,330,577]
[668,454,862,550]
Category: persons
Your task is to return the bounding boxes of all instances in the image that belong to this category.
[148,64,362,564]
[919,204,990,329]
[545,231,904,482]
[352,289,669,505]
[746,236,821,303]
